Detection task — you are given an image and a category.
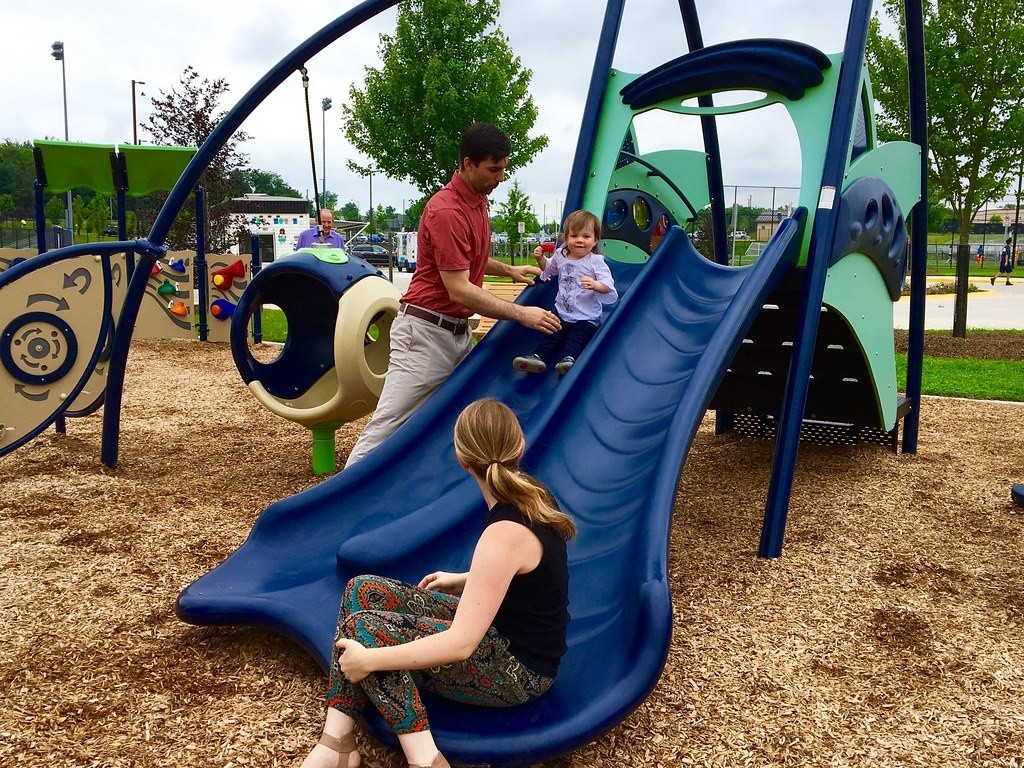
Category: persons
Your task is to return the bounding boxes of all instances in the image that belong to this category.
[1008,229,1014,239]
[512,210,621,373]
[299,395,570,768]
[976,246,985,265]
[347,122,562,471]
[295,208,345,252]
[947,245,955,263]
[991,237,1015,285]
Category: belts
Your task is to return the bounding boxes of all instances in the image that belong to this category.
[399,302,469,336]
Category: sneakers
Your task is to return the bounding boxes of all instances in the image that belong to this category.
[514,355,546,373]
[556,356,575,373]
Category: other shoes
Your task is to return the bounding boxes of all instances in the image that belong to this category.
[1006,282,1013,285]
[991,278,994,285]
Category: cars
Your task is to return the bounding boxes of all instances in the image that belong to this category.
[730,230,748,240]
[346,245,396,267]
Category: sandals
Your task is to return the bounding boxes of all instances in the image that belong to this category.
[319,731,366,768]
[408,752,452,768]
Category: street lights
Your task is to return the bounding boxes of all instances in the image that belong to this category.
[300,68,322,223]
[131,79,145,145]
[321,94,333,206]
[51,41,73,230]
[368,163,375,240]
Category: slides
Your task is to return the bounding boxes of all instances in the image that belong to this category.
[174,217,797,767]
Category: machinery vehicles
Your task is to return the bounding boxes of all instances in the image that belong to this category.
[394,231,419,273]
